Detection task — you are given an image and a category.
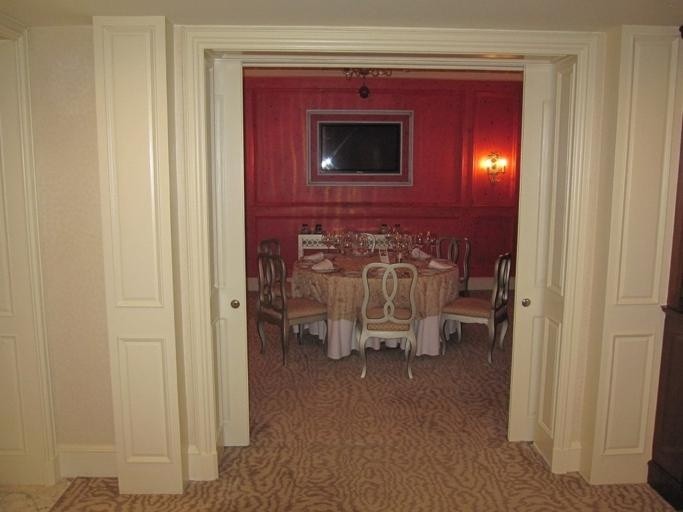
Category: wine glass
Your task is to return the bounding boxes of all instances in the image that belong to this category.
[321,230,337,254]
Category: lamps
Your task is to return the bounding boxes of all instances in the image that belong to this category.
[479,150,508,185]
[341,66,393,99]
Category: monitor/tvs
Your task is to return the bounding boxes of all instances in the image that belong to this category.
[316,120,403,175]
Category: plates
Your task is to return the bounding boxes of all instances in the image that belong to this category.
[311,267,338,273]
[439,264,453,271]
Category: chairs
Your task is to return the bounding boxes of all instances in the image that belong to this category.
[254,234,513,382]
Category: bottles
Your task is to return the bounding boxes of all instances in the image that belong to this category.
[417,231,431,244]
[301,224,322,234]
[380,224,400,234]
